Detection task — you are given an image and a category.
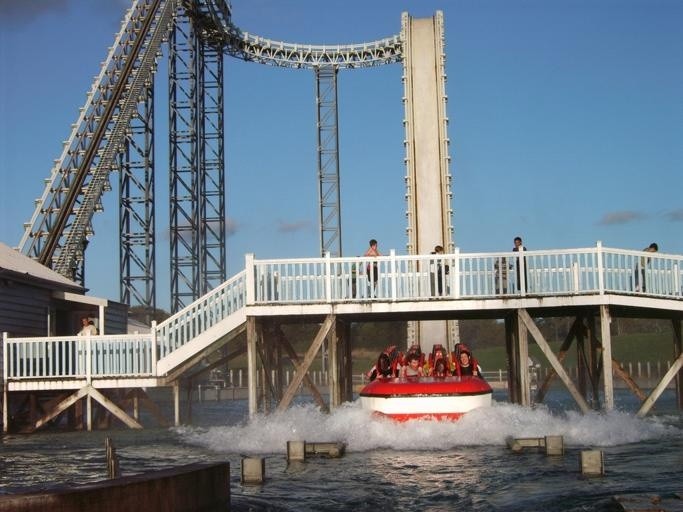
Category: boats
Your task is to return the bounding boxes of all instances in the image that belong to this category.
[357,375,494,421]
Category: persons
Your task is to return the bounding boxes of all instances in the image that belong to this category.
[365,239,383,298]
[513,237,527,293]
[430,246,449,296]
[495,257,513,294]
[352,256,363,298]
[635,243,658,292]
[76,317,98,336]
[366,343,481,380]
